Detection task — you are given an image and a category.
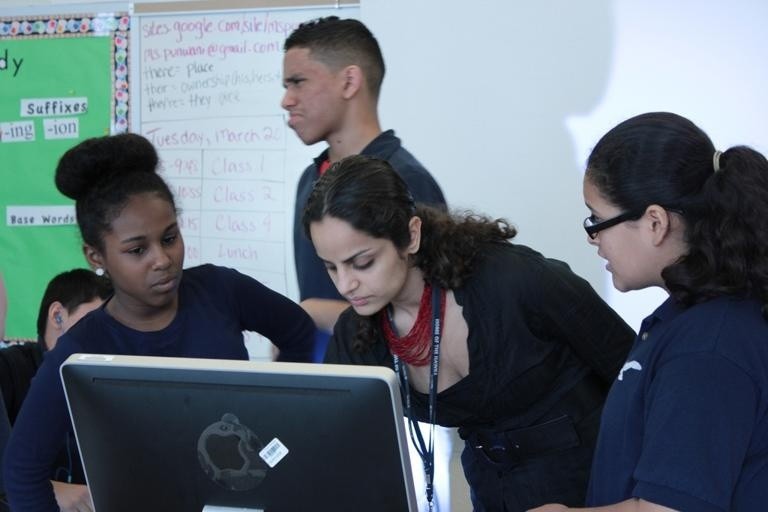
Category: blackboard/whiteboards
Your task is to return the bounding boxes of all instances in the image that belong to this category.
[131,0,768,364]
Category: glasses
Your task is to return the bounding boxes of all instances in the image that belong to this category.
[584,208,644,240]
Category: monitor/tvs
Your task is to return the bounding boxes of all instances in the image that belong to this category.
[58,353,419,512]
